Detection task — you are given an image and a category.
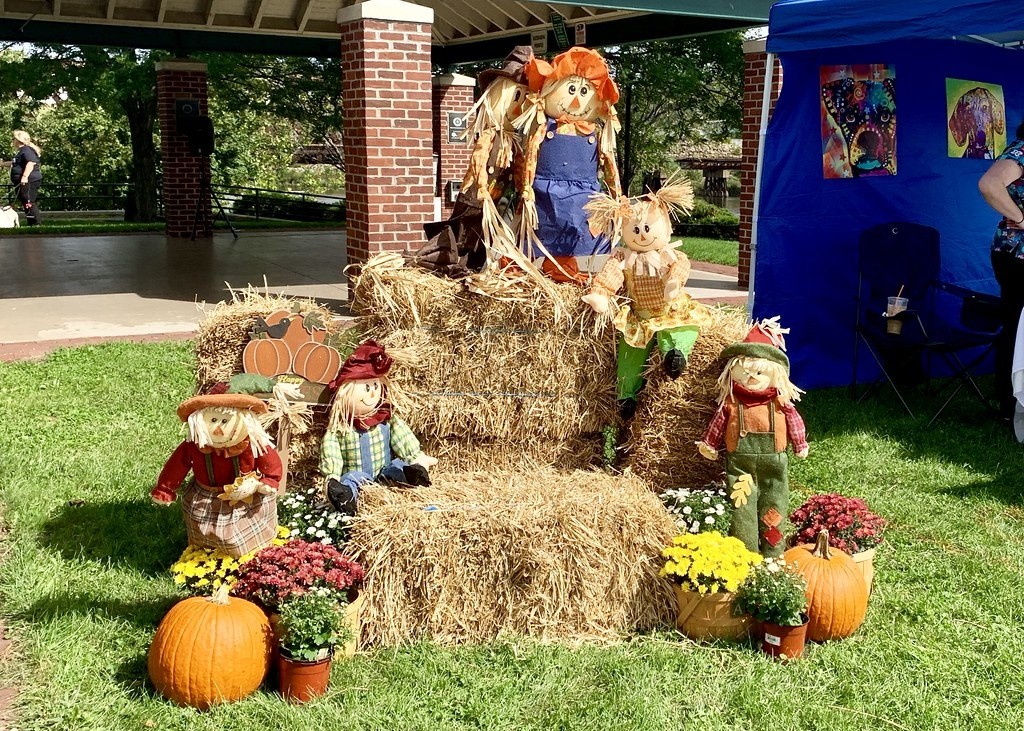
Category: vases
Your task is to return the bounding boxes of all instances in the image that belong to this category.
[672,584,749,641]
[785,533,874,596]
[268,590,363,664]
[754,613,810,660]
[278,638,333,707]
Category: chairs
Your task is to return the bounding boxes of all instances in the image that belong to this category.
[850,221,1001,431]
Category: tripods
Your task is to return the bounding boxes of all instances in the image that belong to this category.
[191,155,238,241]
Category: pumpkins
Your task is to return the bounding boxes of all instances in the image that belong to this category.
[147,583,276,708]
[779,528,867,643]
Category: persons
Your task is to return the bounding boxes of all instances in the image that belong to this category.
[415,46,533,278]
[0,129,43,225]
[979,123,1024,426]
[520,47,624,283]
[702,322,808,560]
[319,340,439,515]
[582,167,699,418]
[149,394,285,556]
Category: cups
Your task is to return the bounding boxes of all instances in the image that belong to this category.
[886,297,909,335]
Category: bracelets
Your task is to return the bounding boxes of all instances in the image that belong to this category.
[23,175,29,177]
[1016,211,1024,224]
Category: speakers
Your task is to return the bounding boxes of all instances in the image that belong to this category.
[190,116,214,156]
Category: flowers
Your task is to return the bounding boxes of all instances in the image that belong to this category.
[169,524,290,597]
[790,492,888,555]
[659,488,734,538]
[736,555,808,625]
[275,585,363,664]
[232,538,366,614]
[274,487,350,544]
[658,530,765,596]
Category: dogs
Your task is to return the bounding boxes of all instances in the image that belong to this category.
[0,205,21,229]
